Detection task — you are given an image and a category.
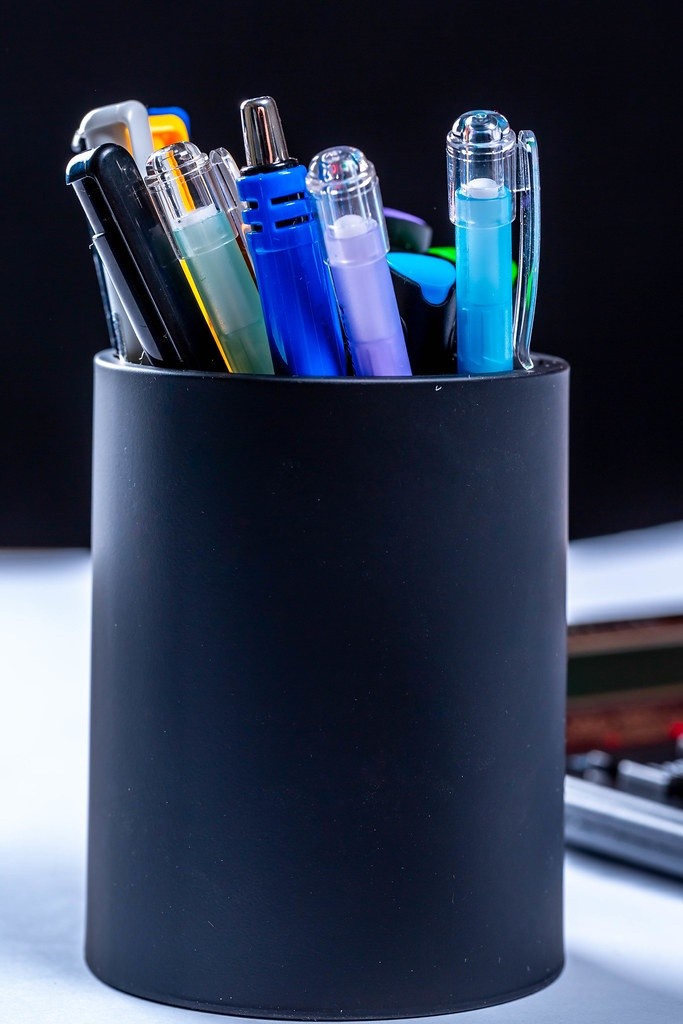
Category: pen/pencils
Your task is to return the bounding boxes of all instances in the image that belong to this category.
[69,101,274,380]
[379,205,457,374]
[308,145,415,378]
[445,107,542,374]
[234,95,355,378]
